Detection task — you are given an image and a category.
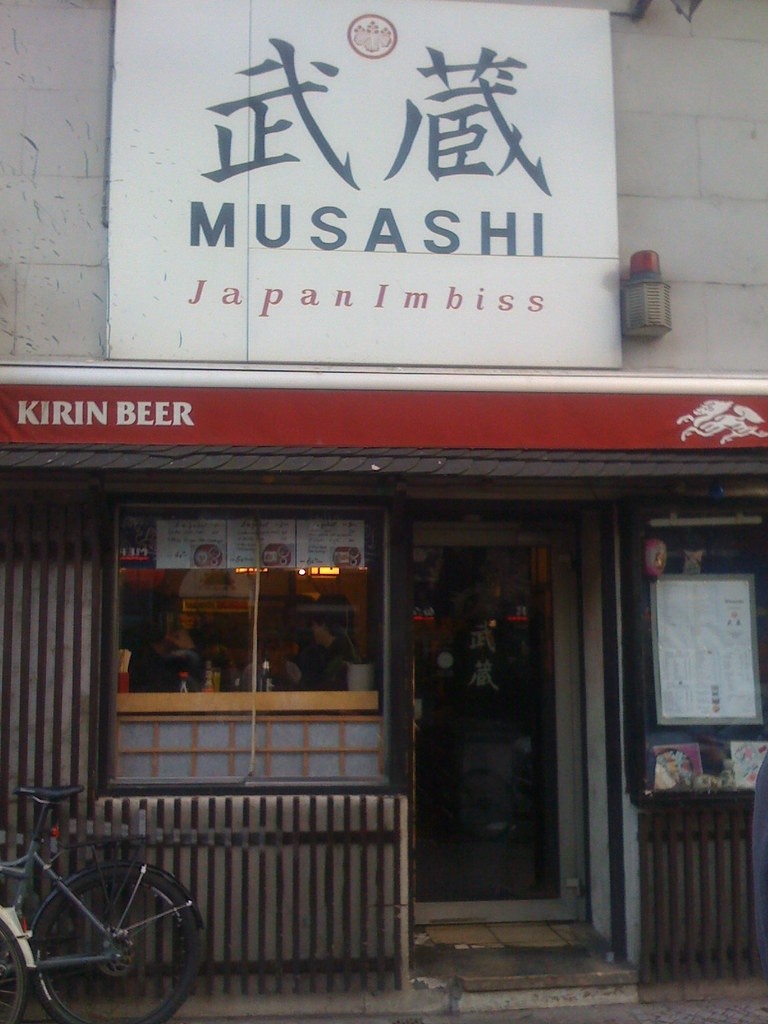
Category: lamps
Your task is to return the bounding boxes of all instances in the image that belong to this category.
[622,248,674,339]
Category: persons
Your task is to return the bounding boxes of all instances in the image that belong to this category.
[419,592,531,851]
[118,591,363,692]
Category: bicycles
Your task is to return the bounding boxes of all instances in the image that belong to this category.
[0,785,209,1024]
[0,903,39,1024]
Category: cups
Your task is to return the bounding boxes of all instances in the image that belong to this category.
[683,549,702,574]
[346,664,374,691]
[118,672,130,694]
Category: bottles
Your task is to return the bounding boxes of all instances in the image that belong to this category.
[257,656,273,692]
[176,672,189,692]
[200,660,215,692]
